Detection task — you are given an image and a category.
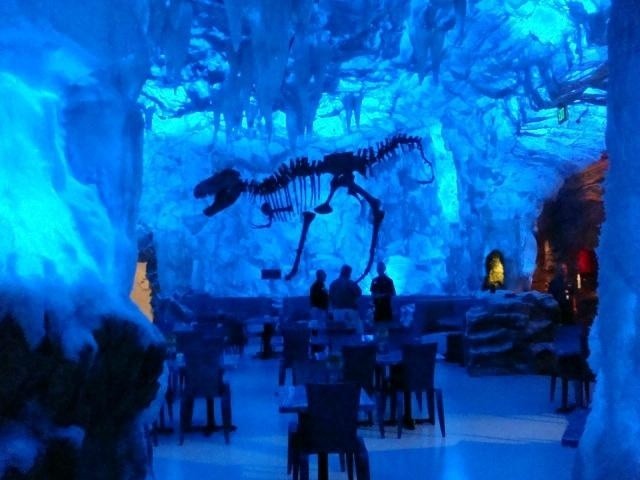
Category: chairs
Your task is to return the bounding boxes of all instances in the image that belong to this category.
[152,303,595,479]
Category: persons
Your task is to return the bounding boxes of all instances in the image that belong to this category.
[330,265,362,309]
[368,262,397,322]
[311,269,329,352]
[547,261,578,326]
